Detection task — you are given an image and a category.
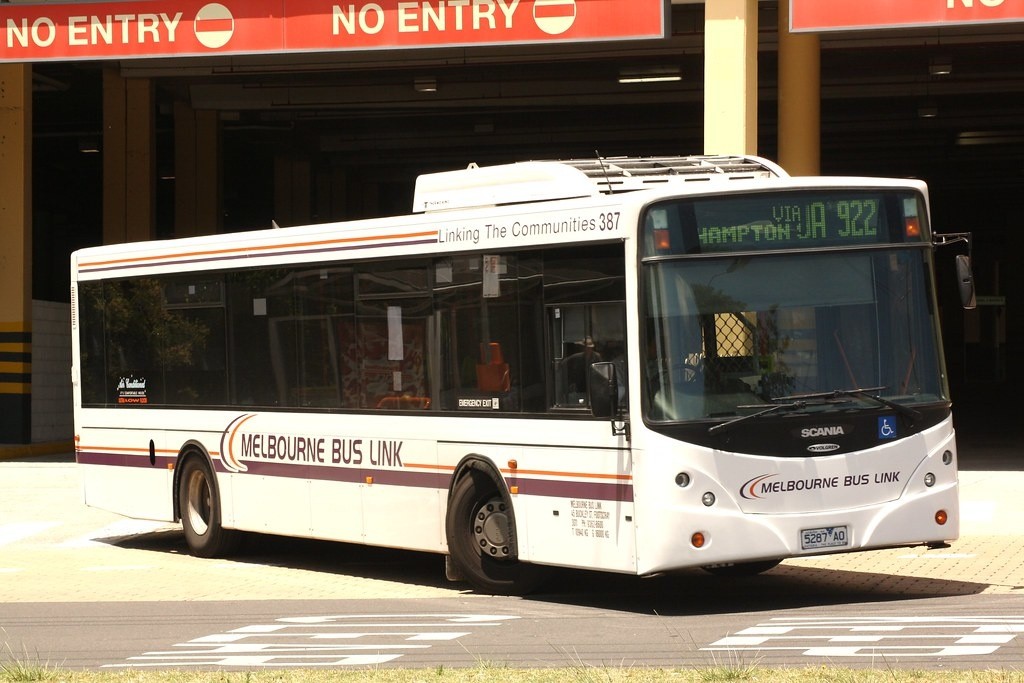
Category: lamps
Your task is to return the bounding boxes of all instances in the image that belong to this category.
[414,82,436,92]
[930,65,951,75]
[618,76,682,82]
[919,107,936,117]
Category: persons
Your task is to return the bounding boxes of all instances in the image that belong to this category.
[748,326,778,399]
[774,331,799,399]
[460,342,483,388]
[557,334,601,404]
[602,323,668,416]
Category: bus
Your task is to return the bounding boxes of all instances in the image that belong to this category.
[68,151,978,595]
